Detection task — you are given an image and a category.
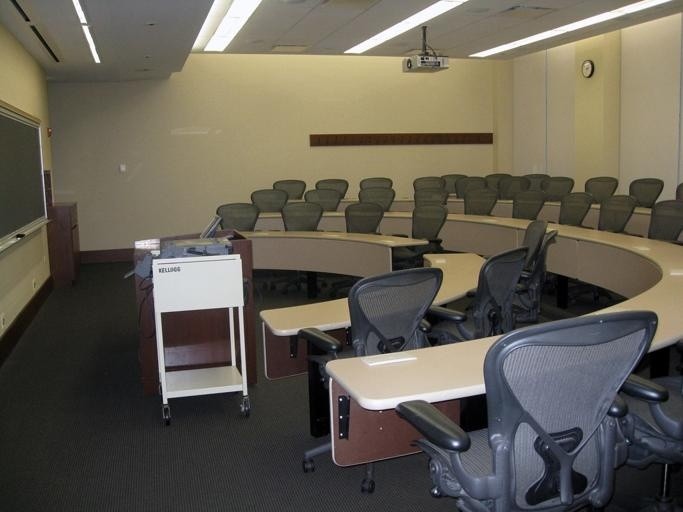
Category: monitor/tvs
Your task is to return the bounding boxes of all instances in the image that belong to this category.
[199,215,222,238]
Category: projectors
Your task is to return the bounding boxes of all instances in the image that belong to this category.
[402,56,449,73]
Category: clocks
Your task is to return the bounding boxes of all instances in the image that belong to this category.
[580,60,594,79]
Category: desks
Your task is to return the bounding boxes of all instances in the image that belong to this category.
[259,252,487,437]
[254,211,682,468]
[133,229,258,426]
[286,198,682,242]
[232,228,428,299]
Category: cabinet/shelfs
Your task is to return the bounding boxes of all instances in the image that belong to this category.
[47,202,81,290]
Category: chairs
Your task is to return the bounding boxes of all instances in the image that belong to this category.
[584,176,618,203]
[496,176,531,200]
[621,374,681,511]
[359,177,393,189]
[393,309,658,511]
[392,204,448,268]
[345,201,384,234]
[485,173,511,192]
[250,189,288,211]
[597,195,636,233]
[216,202,260,232]
[546,177,574,194]
[427,245,529,345]
[413,187,448,207]
[297,266,443,389]
[440,174,468,193]
[454,176,487,199]
[272,179,306,201]
[304,189,340,211]
[523,174,550,192]
[628,178,664,208]
[463,188,497,216]
[511,190,546,220]
[511,230,558,323]
[412,176,445,190]
[357,186,395,212]
[280,202,323,231]
[314,178,348,199]
[519,219,548,267]
[558,192,593,226]
[648,200,682,240]
